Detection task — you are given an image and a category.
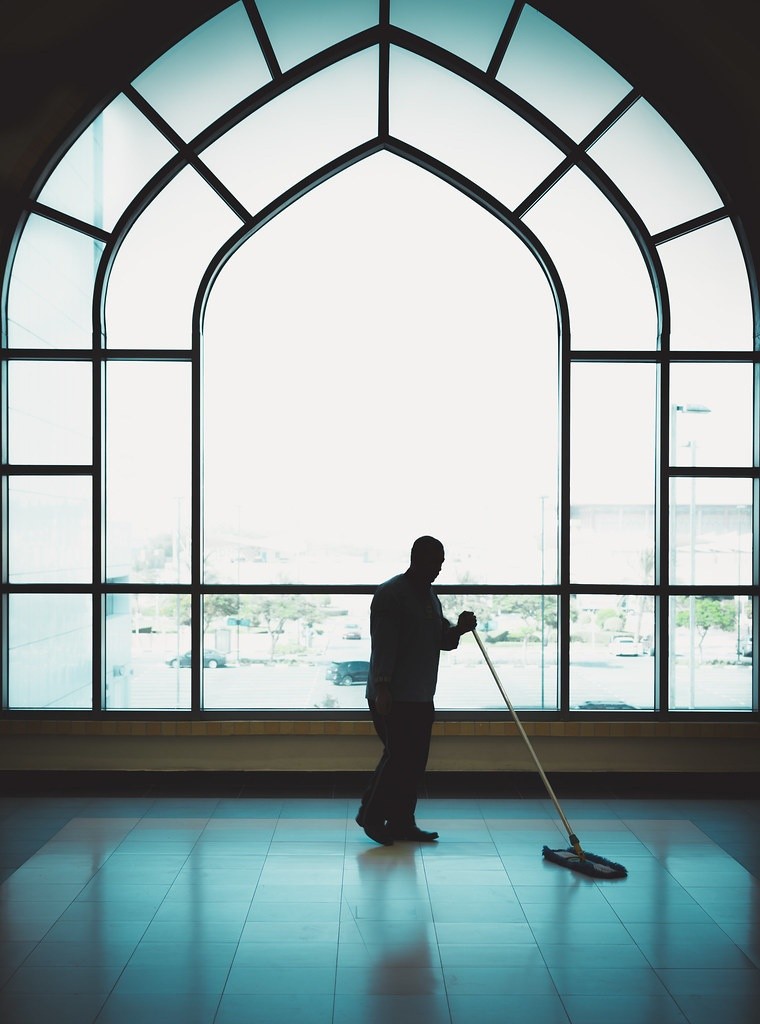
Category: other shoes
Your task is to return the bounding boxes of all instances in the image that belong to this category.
[355,811,393,846]
[386,816,439,841]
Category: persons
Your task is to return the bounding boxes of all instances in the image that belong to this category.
[354,536,477,848]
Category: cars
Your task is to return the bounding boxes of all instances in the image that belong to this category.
[164,648,227,669]
[342,631,362,640]
[734,634,752,657]
[608,636,639,656]
[324,658,371,688]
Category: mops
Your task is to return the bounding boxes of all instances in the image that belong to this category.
[471,624,630,881]
